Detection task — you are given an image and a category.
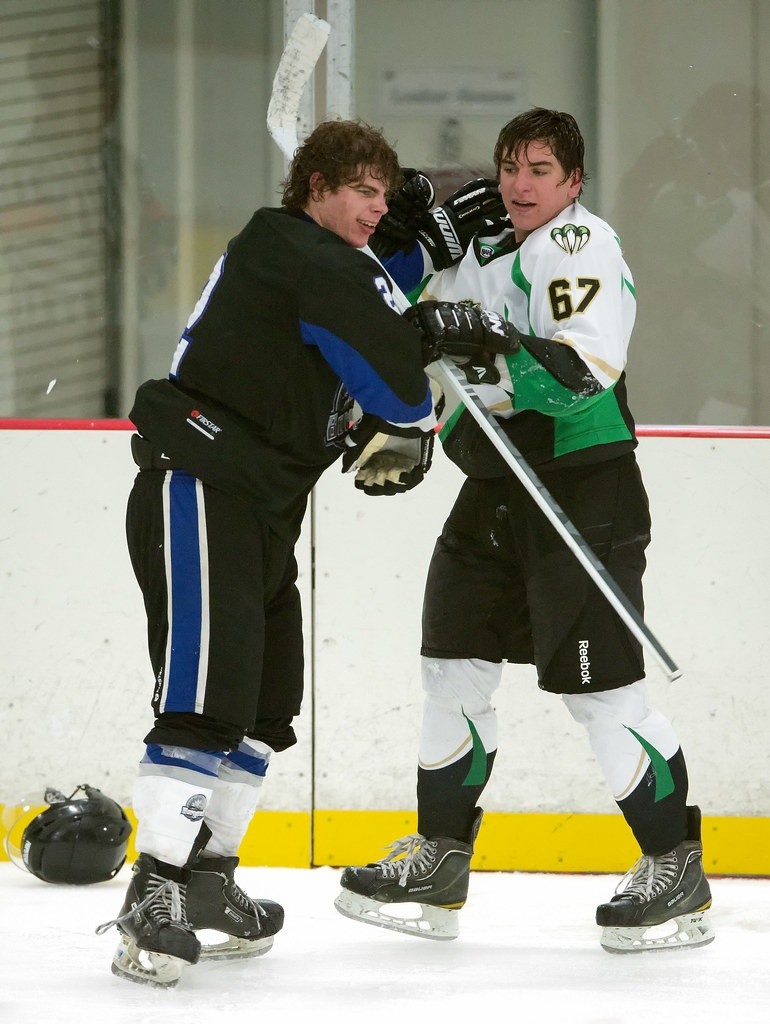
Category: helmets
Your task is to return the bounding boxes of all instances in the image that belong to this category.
[5,783,132,884]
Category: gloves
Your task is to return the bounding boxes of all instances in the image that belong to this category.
[369,167,434,260]
[403,301,522,384]
[342,372,447,496]
[425,176,513,274]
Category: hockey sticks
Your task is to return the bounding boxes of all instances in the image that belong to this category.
[263,9,686,683]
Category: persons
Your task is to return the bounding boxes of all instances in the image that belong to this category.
[328,107,728,951]
[109,115,514,991]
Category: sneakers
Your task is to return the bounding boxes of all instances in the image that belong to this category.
[595,805,718,951]
[334,806,485,940]
[111,852,200,989]
[186,857,285,961]
